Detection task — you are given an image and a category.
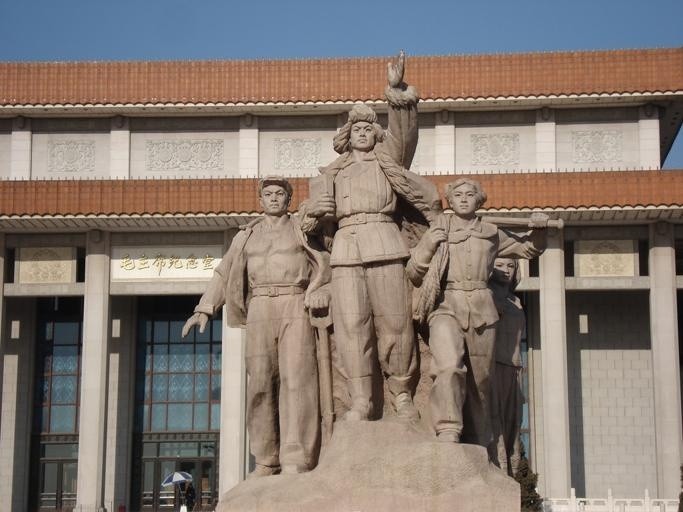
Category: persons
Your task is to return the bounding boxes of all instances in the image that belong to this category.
[302,48,445,422]
[185,483,195,506]
[182,176,334,475]
[489,257,525,478]
[407,177,551,441]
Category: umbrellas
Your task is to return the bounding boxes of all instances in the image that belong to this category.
[161,472,192,504]
